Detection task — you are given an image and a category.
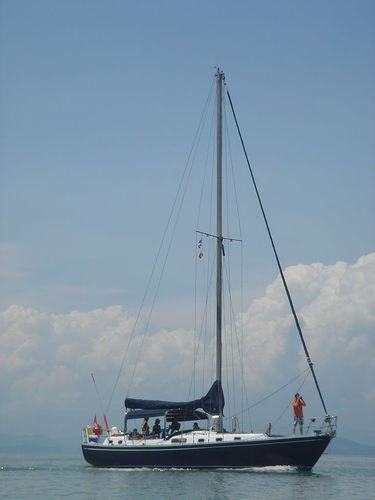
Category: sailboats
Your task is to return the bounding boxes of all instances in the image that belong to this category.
[80,64,339,472]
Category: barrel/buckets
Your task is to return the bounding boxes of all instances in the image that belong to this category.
[88,435,98,443]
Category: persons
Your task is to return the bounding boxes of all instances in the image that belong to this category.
[153,419,160,433]
[193,423,200,430]
[170,421,180,433]
[132,428,140,439]
[141,417,149,434]
[292,393,306,436]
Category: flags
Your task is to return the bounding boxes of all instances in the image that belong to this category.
[198,239,203,258]
